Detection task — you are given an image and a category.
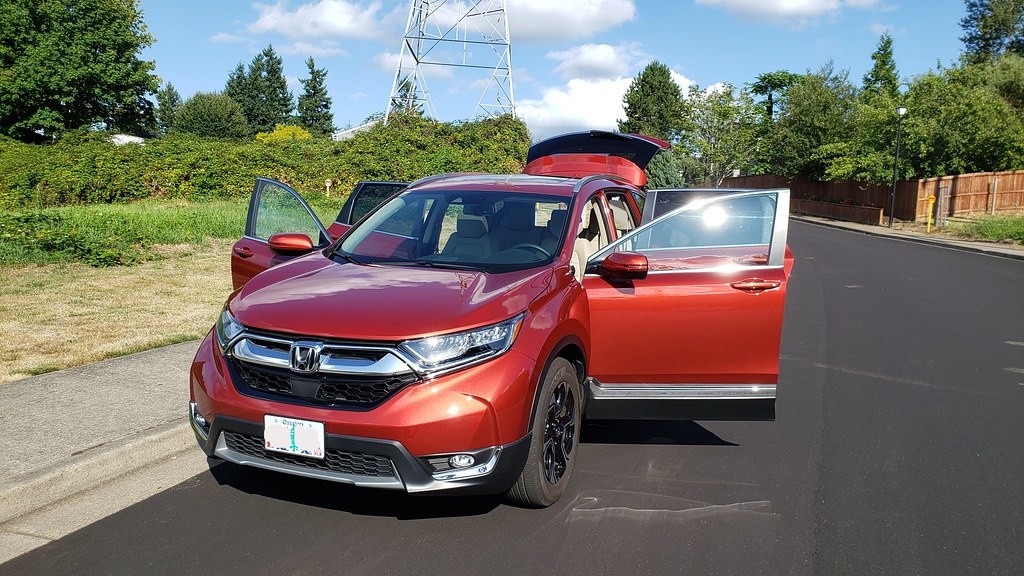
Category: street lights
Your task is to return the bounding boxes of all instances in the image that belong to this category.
[889,105,908,228]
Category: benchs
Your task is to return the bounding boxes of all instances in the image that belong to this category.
[493,203,637,258]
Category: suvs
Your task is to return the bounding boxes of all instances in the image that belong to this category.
[186,129,795,508]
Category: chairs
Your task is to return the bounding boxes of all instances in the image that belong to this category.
[440,210,500,259]
[538,209,596,282]
